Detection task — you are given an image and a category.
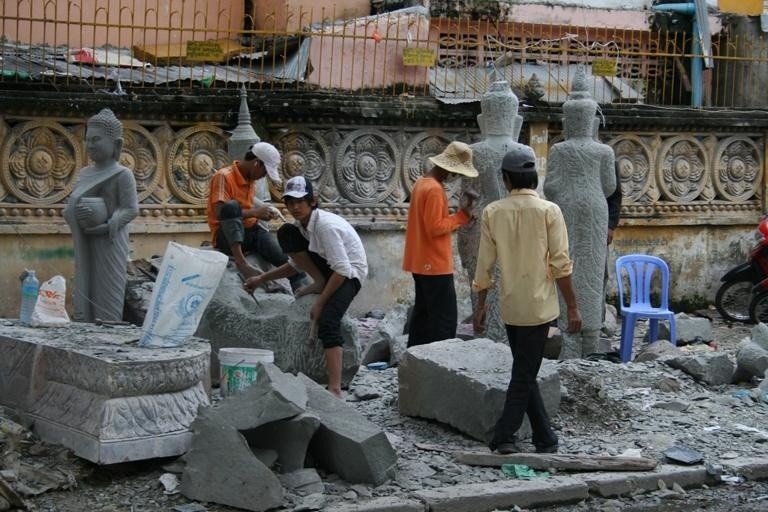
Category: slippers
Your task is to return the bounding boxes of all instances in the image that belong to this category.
[250,263,275,289]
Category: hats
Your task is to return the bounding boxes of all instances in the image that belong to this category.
[501,146,538,173]
[249,141,282,183]
[279,176,313,199]
[428,141,479,178]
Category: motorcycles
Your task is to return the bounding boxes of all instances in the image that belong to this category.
[713,215,768,327]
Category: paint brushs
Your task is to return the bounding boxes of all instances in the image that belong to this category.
[237,273,261,308]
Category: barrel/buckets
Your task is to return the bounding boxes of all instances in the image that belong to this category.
[218,346,273,401]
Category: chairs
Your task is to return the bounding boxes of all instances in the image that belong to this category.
[613,253,677,363]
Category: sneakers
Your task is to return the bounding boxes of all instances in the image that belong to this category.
[537,435,560,452]
[489,438,521,454]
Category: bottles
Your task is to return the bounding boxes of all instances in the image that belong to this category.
[18,269,40,324]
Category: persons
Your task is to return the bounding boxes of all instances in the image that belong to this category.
[542,66,617,335]
[62,108,140,323]
[460,81,535,343]
[599,161,622,337]
[240,174,369,408]
[471,148,582,455]
[405,141,478,347]
[205,142,315,296]
